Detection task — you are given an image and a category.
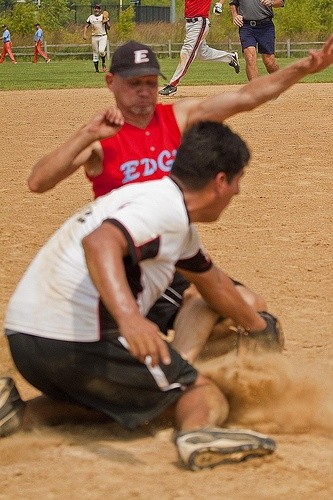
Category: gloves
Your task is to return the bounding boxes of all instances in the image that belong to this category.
[93,3,101,9]
[212,3,222,16]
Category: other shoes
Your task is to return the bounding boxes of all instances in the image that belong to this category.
[47,59,51,64]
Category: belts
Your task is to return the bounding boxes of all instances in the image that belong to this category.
[186,18,198,23]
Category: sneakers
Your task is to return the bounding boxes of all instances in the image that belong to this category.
[158,85,177,97]
[173,426,275,471]
[0,377,25,438]
[228,52,240,74]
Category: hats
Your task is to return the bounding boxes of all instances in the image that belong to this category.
[2,25,7,27]
[34,23,40,26]
[110,40,168,81]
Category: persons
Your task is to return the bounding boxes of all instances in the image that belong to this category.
[0,121,285,471]
[32,23,51,63]
[28,34,333,363]
[0,24,17,64]
[83,3,110,72]
[159,0,241,96]
[229,0,284,82]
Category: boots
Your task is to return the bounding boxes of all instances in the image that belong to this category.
[94,61,99,72]
[101,56,106,66]
